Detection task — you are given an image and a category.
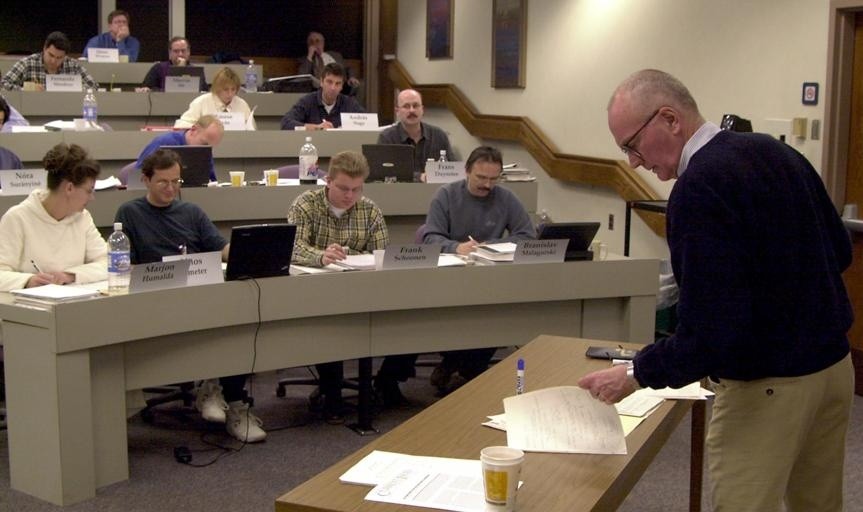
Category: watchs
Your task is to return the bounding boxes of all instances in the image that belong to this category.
[625,362,642,391]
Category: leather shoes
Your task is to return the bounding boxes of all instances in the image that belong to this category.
[322,393,345,425]
[373,379,411,408]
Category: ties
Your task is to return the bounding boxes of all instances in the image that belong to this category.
[317,55,323,68]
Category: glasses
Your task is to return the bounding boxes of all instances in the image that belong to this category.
[78,185,96,196]
[620,110,658,158]
[150,179,184,189]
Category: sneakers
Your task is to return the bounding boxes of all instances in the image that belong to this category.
[431,366,445,393]
[446,373,465,391]
[226,401,266,443]
[195,382,230,422]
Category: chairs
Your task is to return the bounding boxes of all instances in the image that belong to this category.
[276,364,387,411]
[138,386,255,435]
[413,224,501,387]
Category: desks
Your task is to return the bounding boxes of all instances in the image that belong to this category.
[0,59,267,96]
[274,333,707,510]
[0,246,660,506]
[1,179,539,245]
[1,85,305,134]
[1,129,380,179]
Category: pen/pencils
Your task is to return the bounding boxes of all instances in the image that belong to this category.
[338,238,355,250]
[468,235,472,239]
[32,260,42,274]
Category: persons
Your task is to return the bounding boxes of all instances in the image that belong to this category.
[0,142,147,422]
[0,96,24,169]
[378,89,457,184]
[296,31,361,96]
[83,10,140,62]
[136,37,211,92]
[288,151,415,424]
[114,149,268,444]
[577,69,854,511]
[415,146,537,392]
[1,32,100,90]
[280,63,367,131]
[174,65,256,131]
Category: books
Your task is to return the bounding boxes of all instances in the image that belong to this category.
[10,283,97,309]
[291,253,375,276]
[470,241,515,261]
[500,163,535,182]
[44,119,74,131]
[437,252,494,267]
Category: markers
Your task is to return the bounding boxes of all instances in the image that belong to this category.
[516,359,525,394]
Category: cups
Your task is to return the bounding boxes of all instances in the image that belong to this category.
[481,445,524,512]
[229,171,244,187]
[264,170,278,186]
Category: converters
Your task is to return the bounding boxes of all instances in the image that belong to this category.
[173,444,194,466]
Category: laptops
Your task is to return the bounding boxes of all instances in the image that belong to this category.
[536,221,600,260]
[223,224,297,280]
[162,145,212,187]
[163,65,204,94]
[361,143,414,182]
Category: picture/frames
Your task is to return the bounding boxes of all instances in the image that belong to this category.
[375,0,398,126]
[490,1,527,93]
[424,2,453,63]
[800,81,819,105]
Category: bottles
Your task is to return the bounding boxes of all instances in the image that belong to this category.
[85,90,98,125]
[248,61,259,93]
[105,222,131,297]
[300,136,320,186]
[439,151,449,163]
